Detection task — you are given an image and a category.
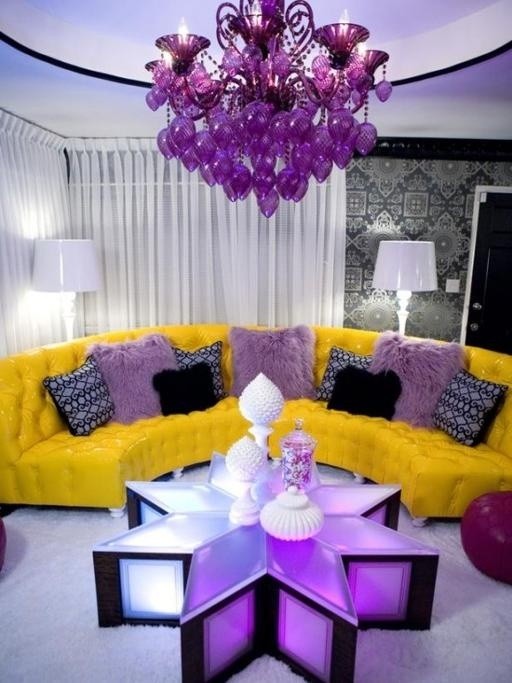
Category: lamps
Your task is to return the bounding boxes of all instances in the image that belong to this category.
[144,0,395,217]
[373,240,440,338]
[237,368,283,470]
[31,236,103,343]
[223,427,262,527]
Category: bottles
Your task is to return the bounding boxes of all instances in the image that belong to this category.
[278,416,319,492]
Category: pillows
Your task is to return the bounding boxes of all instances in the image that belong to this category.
[224,322,319,399]
[40,356,117,434]
[89,333,183,423]
[372,331,467,431]
[326,363,403,419]
[162,340,227,392]
[317,345,376,396]
[432,367,509,450]
[149,360,217,416]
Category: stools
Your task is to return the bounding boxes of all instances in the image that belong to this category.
[462,489,512,586]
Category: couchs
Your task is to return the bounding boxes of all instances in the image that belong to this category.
[0,322,512,526]
